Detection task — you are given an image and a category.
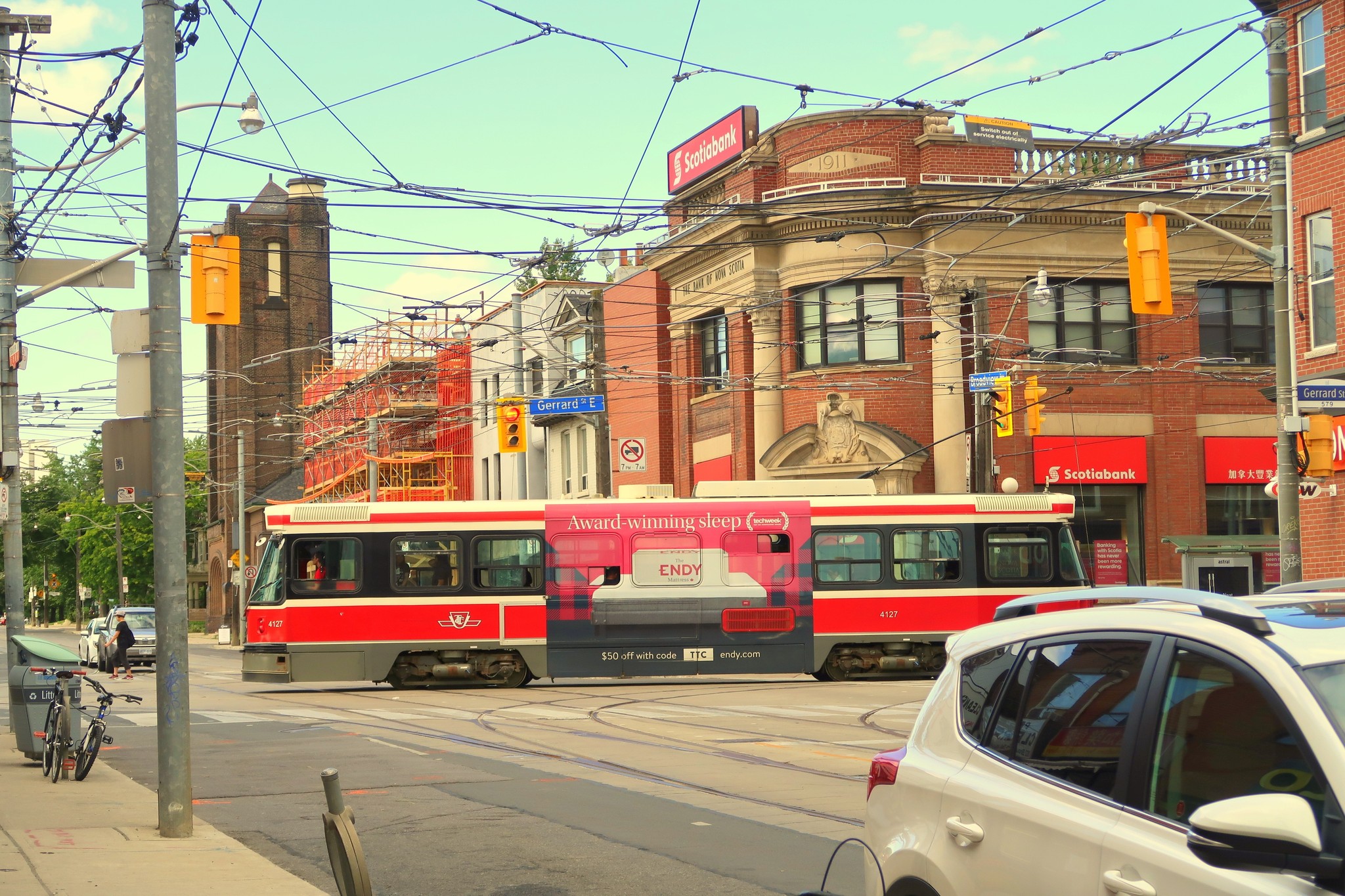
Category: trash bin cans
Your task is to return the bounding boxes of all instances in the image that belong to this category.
[8,635,82,764]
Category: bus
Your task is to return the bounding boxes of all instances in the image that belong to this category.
[239,385,1094,690]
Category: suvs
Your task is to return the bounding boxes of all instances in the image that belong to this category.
[864,576,1345,896]
[97,603,156,674]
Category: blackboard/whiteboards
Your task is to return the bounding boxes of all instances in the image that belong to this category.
[218,624,230,645]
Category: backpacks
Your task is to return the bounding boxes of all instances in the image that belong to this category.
[125,629,135,646]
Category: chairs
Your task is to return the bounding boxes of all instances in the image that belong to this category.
[521,567,533,587]
[1178,684,1292,802]
[447,576,454,586]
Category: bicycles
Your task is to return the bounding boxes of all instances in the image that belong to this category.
[68,676,142,781]
[30,667,87,783]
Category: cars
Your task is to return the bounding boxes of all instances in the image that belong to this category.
[79,617,141,668]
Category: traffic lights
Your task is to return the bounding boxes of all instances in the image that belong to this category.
[496,398,527,453]
[1023,376,1047,436]
[988,376,1013,438]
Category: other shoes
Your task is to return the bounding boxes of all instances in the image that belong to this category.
[108,673,119,680]
[121,675,133,680]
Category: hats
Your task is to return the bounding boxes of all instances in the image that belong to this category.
[113,610,126,617]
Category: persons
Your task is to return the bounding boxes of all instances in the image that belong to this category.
[431,549,451,586]
[604,567,619,585]
[935,563,954,580]
[104,611,133,680]
[904,565,918,580]
[828,566,844,581]
[305,552,326,590]
[395,553,410,586]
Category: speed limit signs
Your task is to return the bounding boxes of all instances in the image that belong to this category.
[83,588,92,598]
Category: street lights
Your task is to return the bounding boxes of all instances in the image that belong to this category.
[1,91,267,732]
[65,513,124,608]
[273,409,378,503]
[33,522,81,632]
[452,314,611,499]
[974,265,1052,495]
